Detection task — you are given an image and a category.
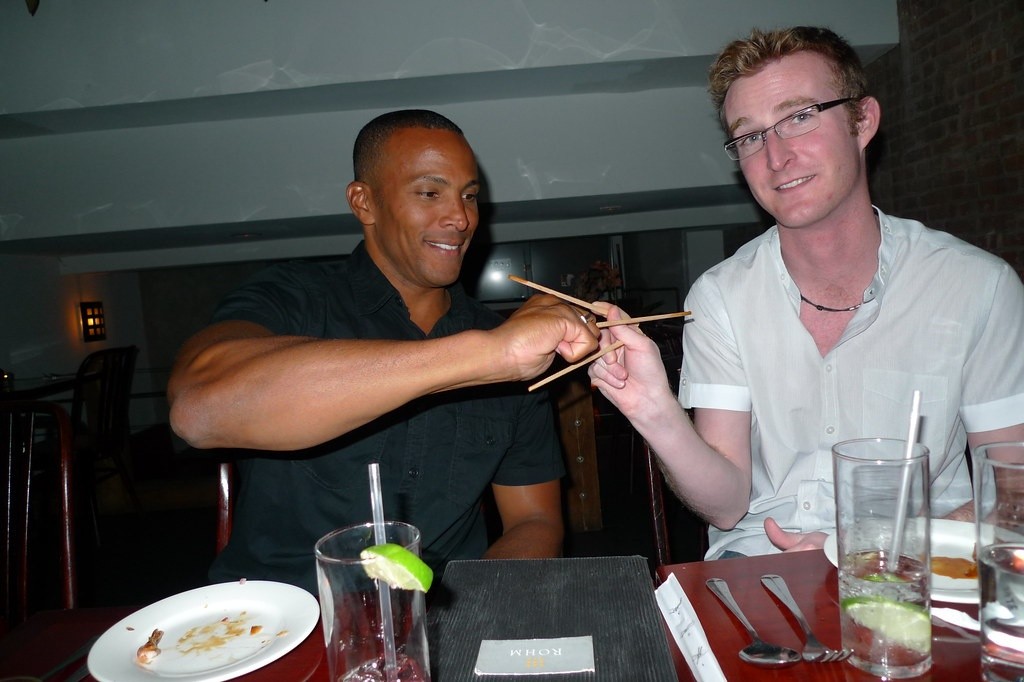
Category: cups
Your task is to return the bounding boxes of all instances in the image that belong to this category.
[313,521,431,682]
[970,441,1024,682]
[831,437,932,679]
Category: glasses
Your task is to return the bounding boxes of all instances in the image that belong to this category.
[725,93,869,160]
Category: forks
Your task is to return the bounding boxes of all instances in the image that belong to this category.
[759,573,855,663]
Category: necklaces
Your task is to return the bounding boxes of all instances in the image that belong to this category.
[800,295,861,311]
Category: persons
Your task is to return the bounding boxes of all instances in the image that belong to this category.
[586,27,1024,561]
[168,109,600,589]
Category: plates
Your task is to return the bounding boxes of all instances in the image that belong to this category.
[86,580,320,682]
[823,516,1024,604]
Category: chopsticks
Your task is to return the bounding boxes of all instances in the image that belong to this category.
[507,273,639,393]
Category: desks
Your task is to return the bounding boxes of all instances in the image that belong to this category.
[0,550,983,682]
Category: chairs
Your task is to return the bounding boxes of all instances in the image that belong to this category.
[635,323,710,567]
[0,344,140,644]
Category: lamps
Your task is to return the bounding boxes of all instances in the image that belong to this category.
[79,301,106,343]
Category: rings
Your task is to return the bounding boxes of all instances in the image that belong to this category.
[580,314,590,322]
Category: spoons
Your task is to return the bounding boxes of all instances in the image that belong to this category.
[705,577,802,664]
